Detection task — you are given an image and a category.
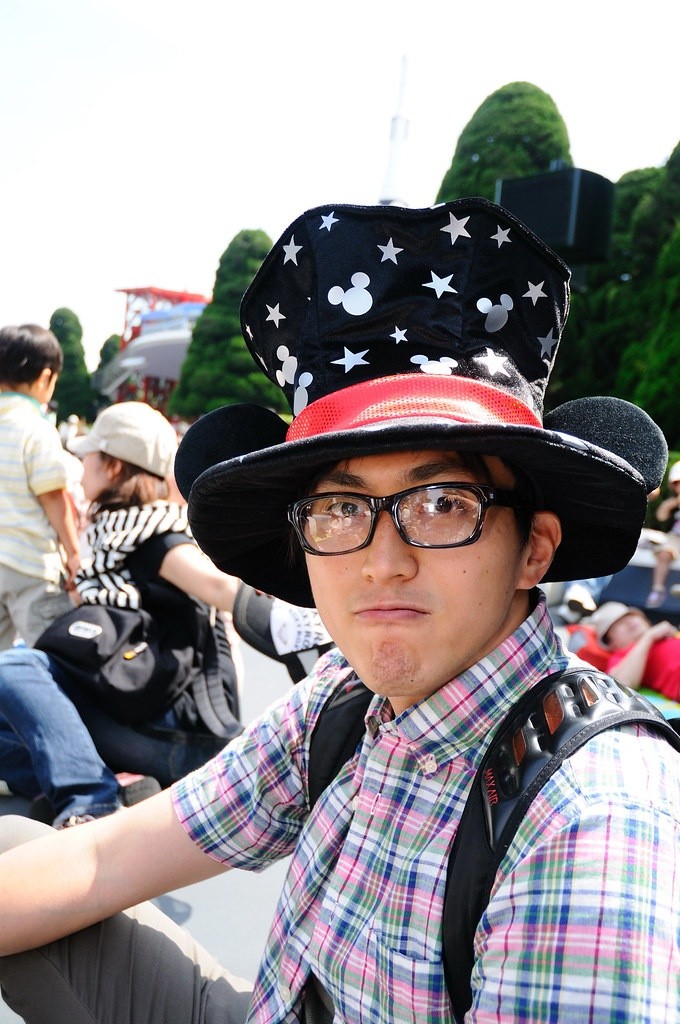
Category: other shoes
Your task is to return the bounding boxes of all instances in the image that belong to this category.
[29,771,162,826]
[557,584,598,624]
[645,586,668,609]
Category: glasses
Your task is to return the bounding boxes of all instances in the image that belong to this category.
[286,480,537,557]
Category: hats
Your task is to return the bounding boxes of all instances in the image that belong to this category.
[578,601,645,643]
[669,460,680,483]
[174,198,668,609]
[66,401,179,479]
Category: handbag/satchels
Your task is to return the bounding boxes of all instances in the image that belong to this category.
[32,532,246,740]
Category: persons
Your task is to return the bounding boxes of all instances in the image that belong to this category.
[592,601,680,703]
[0,399,242,839]
[0,196,680,1024]
[557,574,613,624]
[0,323,81,650]
[645,461,680,608]
